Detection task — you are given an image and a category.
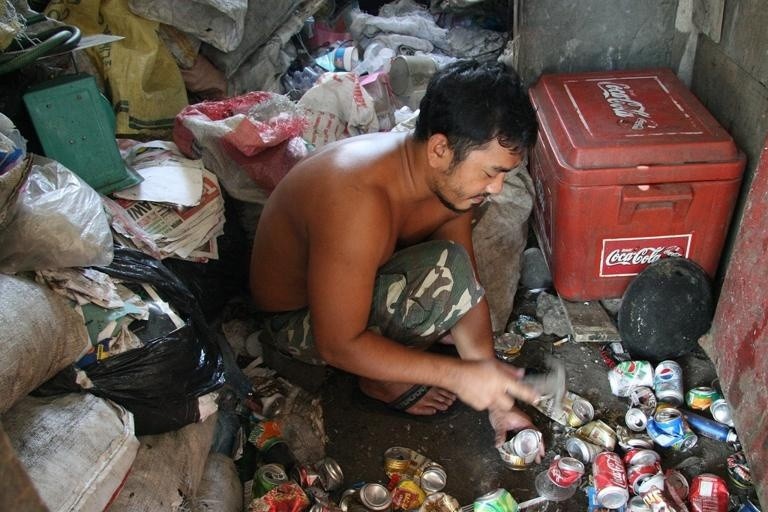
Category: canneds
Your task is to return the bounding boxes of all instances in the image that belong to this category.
[238,374,345,512]
[473,487,518,512]
[497,426,543,471]
[616,386,698,453]
[726,450,754,489]
[592,451,629,510]
[565,419,617,466]
[686,474,730,511]
[492,315,543,363]
[339,445,460,512]
[547,455,585,489]
[533,389,594,427]
[622,447,690,511]
[685,387,736,428]
[653,360,684,408]
[607,360,655,397]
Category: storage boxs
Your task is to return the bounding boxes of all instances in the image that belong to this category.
[528,67,749,302]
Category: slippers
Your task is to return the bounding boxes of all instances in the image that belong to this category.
[356,377,464,422]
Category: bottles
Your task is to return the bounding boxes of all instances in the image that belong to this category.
[248,415,297,475]
[664,405,739,444]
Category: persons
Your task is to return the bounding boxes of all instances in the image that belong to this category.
[248,60,545,464]
